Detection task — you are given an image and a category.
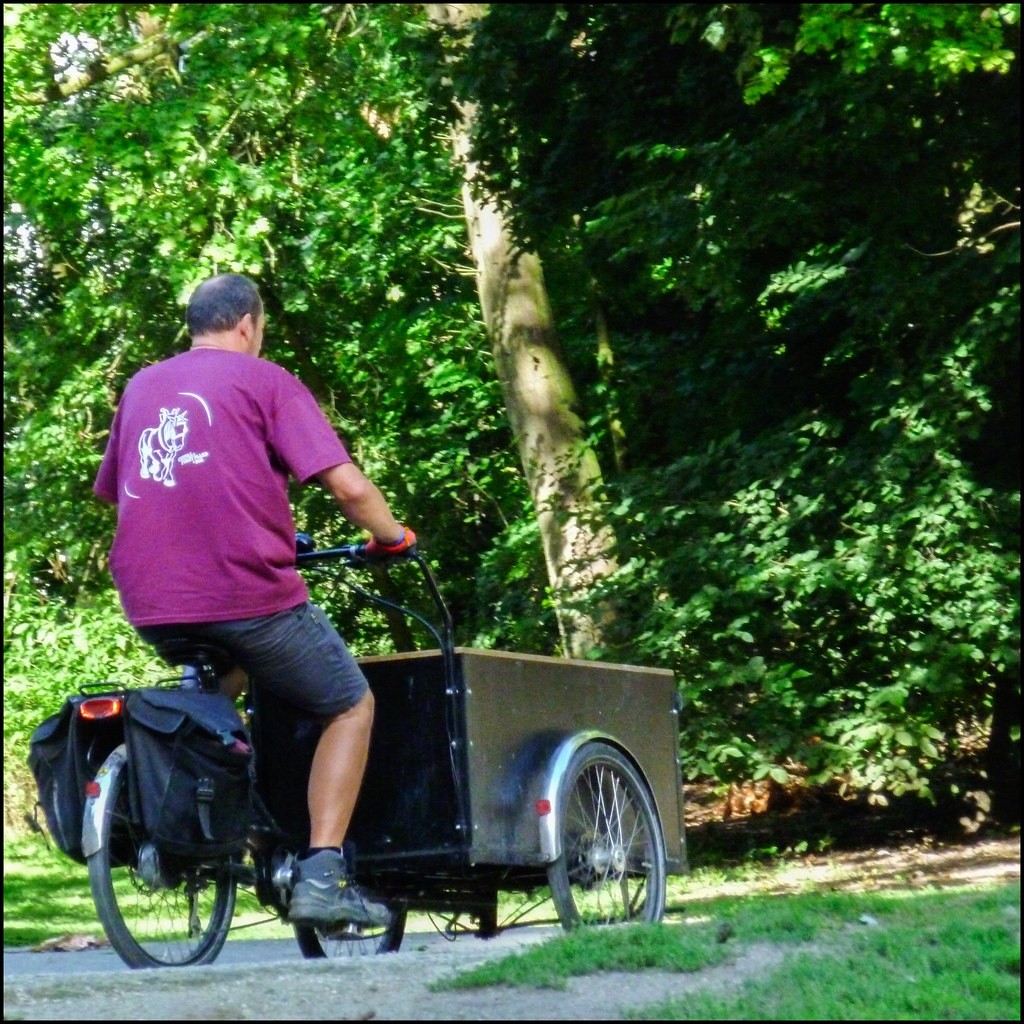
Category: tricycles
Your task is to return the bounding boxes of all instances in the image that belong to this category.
[69,536,689,969]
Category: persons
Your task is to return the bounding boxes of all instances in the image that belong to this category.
[94,274,416,922]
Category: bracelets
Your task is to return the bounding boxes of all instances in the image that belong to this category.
[375,529,404,546]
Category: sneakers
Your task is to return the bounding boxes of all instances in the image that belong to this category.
[289,883,396,928]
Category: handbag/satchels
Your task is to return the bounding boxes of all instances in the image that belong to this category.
[120,687,256,857]
[23,691,139,868]
